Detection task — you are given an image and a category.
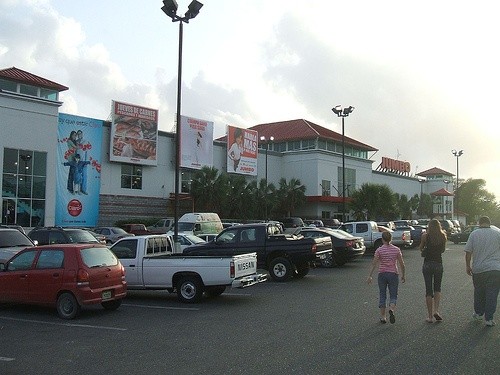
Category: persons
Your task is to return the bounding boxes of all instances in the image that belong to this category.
[420,219,447,322]
[464,216,500,326]
[367,231,405,324]
[228,128,244,172]
[61,129,92,195]
[195,132,203,164]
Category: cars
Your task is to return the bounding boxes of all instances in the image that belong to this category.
[283,227,366,268]
[0,245,128,319]
[442,220,462,236]
[260,216,341,234]
[92,227,135,244]
[84,228,107,245]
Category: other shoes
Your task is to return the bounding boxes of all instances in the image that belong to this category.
[471,311,484,320]
[486,319,496,326]
[434,313,442,320]
[379,318,386,323]
[388,309,396,323]
[428,318,433,323]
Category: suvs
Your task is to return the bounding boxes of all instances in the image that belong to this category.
[0,225,38,261]
[26,226,101,249]
[147,218,177,235]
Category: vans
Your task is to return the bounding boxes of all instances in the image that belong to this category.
[167,213,224,236]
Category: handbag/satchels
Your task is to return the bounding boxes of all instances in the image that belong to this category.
[420,232,430,257]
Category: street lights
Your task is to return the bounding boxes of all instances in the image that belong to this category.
[331,105,354,223]
[259,135,275,224]
[160,0,204,243]
[450,150,463,221]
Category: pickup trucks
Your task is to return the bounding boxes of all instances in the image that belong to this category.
[184,224,335,282]
[107,233,270,302]
[337,221,412,255]
[378,219,430,247]
[123,224,166,236]
